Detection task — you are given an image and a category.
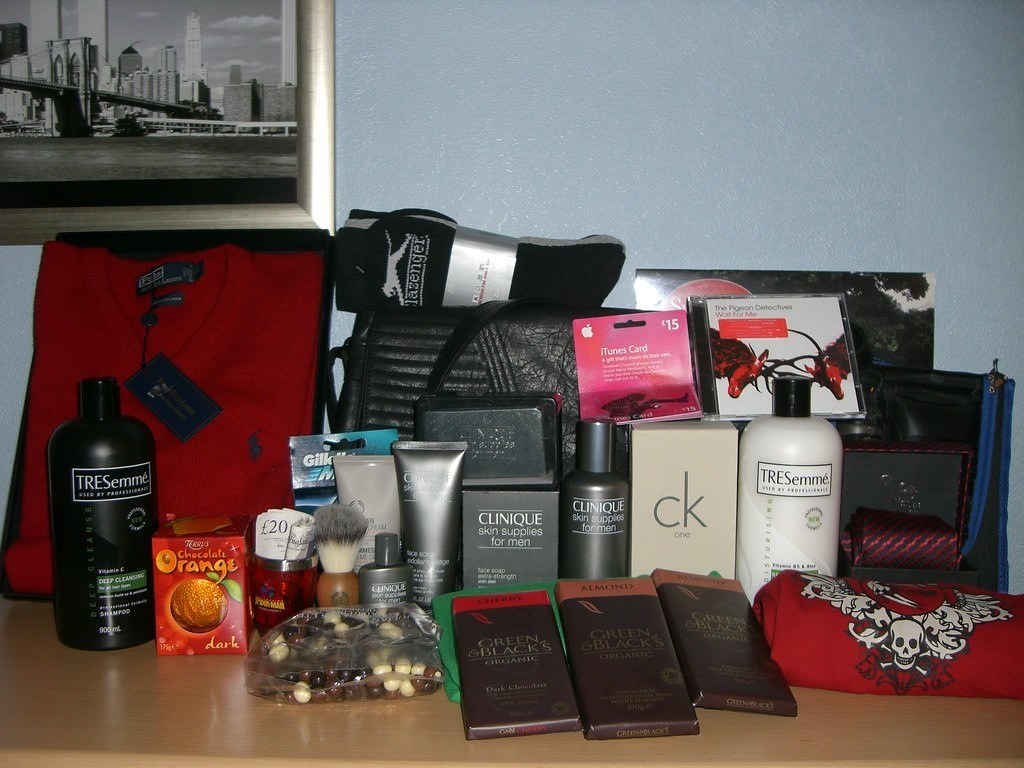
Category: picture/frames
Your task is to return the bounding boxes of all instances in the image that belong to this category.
[0,0,336,248]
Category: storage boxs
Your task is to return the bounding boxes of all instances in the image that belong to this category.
[836,442,990,591]
[554,578,703,741]
[150,510,251,656]
[650,568,799,719]
[628,421,738,580]
[450,590,583,741]
[460,484,559,584]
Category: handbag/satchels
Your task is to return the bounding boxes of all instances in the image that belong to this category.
[325,297,698,454]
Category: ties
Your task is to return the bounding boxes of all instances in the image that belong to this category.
[841,509,959,569]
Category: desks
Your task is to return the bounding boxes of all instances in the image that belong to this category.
[1,587,1024,767]
